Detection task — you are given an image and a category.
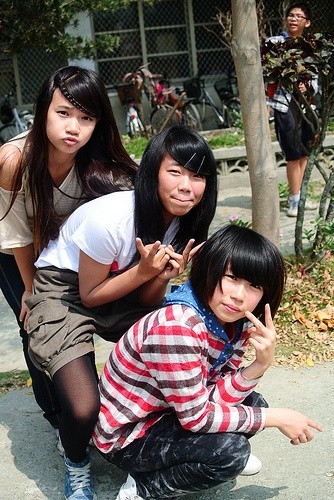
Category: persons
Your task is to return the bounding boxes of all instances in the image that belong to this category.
[24,125,218,500]
[264,3,323,216]
[0,66,139,458]
[90,225,323,500]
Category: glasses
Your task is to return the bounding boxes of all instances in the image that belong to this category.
[288,13,307,20]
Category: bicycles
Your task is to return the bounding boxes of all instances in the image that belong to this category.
[114,78,149,142]
[180,65,243,131]
[144,73,204,137]
[0,83,36,146]
[213,74,277,127]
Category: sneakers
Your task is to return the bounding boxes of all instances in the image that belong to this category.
[62,453,97,500]
[114,472,141,500]
[238,451,263,476]
[287,196,300,217]
[305,198,318,212]
[54,429,67,459]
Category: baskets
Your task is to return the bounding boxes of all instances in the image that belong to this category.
[0,99,14,125]
[213,77,239,101]
[183,78,202,99]
[116,84,142,106]
